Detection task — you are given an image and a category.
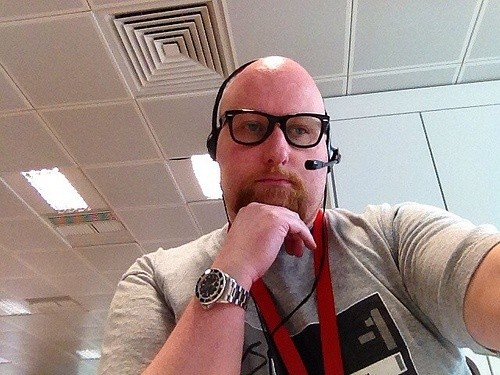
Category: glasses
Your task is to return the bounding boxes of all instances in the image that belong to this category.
[216,107,331,151]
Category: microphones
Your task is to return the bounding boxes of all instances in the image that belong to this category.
[305,159,338,170]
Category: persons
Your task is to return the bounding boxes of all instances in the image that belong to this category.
[101,55,500,375]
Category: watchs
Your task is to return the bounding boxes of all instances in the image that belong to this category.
[194,264,250,310]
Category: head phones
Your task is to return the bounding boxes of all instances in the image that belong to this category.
[207,60,341,172]
